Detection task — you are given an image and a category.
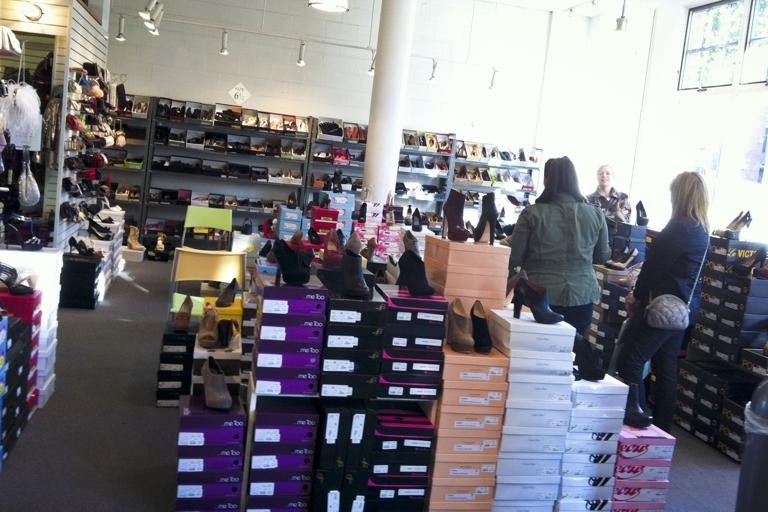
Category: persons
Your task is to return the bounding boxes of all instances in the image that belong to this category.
[586,165,632,224]
[508,156,612,336]
[616,171,711,436]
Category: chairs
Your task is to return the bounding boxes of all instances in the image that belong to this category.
[177,202,232,255]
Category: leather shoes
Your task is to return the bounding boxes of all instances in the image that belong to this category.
[572,334,605,381]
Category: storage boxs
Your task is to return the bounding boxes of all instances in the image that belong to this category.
[0,206,125,470]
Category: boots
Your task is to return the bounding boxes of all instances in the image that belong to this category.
[613,371,653,428]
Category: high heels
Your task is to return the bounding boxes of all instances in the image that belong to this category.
[259,245,435,298]
[0,209,116,294]
[448,298,492,354]
[712,210,753,241]
[615,199,649,225]
[604,246,639,271]
[511,277,566,325]
[733,244,768,280]
[174,278,241,410]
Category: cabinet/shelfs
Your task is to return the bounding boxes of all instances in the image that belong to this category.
[57,53,116,237]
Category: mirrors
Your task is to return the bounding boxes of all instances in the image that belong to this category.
[1,25,59,249]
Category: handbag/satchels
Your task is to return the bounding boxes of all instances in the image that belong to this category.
[645,294,689,330]
[1,52,127,224]
